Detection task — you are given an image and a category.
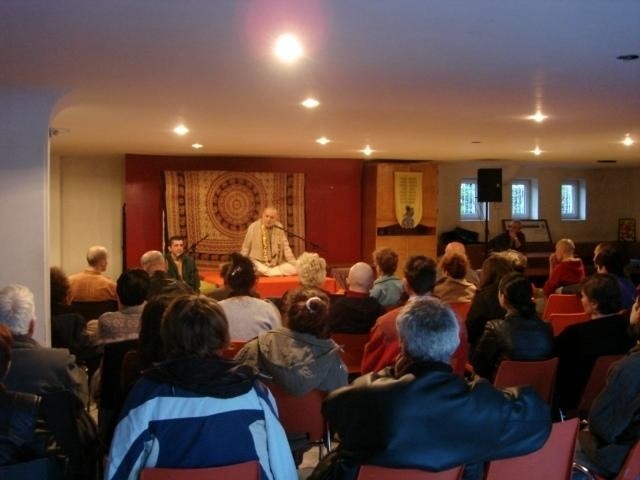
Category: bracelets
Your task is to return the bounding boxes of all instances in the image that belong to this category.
[514,238,518,240]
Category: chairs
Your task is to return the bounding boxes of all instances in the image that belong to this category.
[267,387,331,465]
[487,417,579,480]
[541,292,630,420]
[574,441,638,480]
[2,299,138,479]
[441,302,473,341]
[141,460,260,480]
[219,340,246,359]
[327,335,371,373]
[358,464,461,480]
[494,359,560,404]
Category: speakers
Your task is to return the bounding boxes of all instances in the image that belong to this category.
[477,168,502,202]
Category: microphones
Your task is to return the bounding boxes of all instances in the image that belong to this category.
[176,253,186,259]
[272,222,284,231]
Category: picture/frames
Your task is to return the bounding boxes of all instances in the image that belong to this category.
[502,219,551,245]
[618,219,635,243]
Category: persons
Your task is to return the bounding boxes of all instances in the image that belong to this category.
[241,207,296,266]
[330,240,640,422]
[488,220,527,254]
[1,284,100,479]
[51,236,348,479]
[579,286,640,478]
[320,298,551,478]
